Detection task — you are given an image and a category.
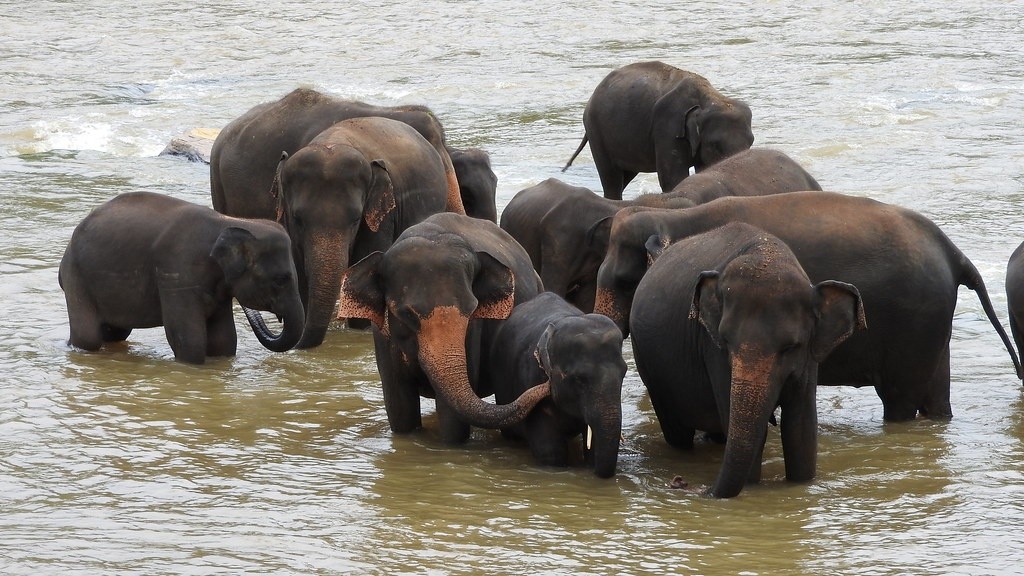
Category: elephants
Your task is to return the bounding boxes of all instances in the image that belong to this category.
[58,192,306,364]
[561,54,752,201]
[209,87,1023,498]
